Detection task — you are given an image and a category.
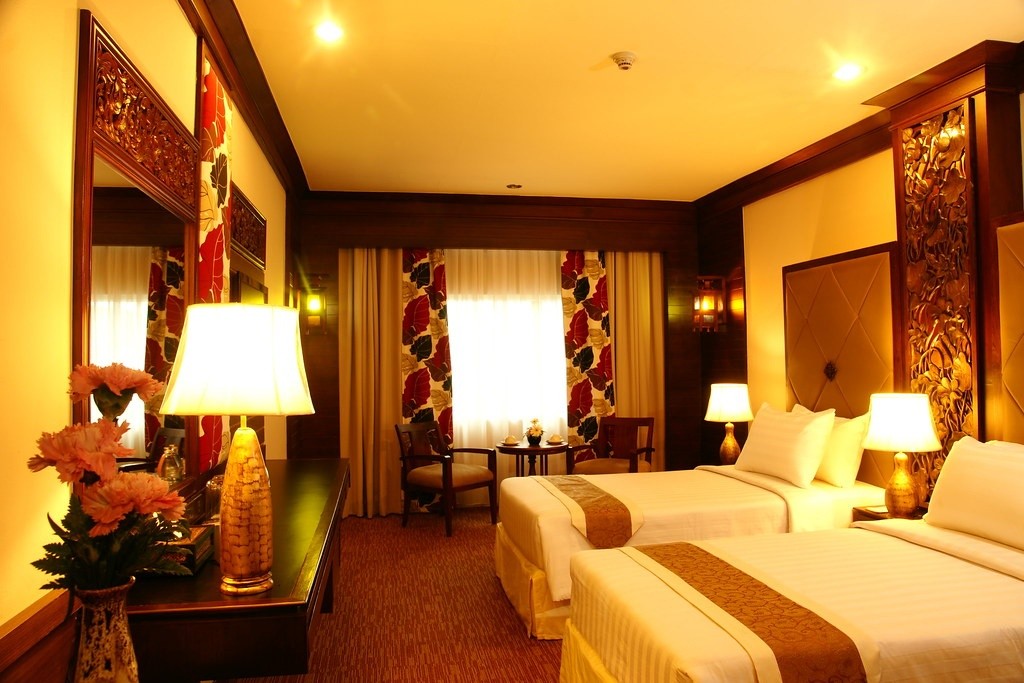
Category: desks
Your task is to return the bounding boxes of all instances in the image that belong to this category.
[496,442,569,477]
[127,459,350,683]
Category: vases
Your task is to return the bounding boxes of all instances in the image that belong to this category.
[527,436,541,446]
[74,575,138,683]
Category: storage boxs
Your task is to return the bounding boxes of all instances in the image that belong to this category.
[140,524,216,576]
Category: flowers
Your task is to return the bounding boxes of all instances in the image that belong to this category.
[27,361,187,633]
[522,418,545,439]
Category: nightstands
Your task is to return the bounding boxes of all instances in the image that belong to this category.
[851,504,930,524]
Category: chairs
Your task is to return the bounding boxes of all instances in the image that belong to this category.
[395,421,498,537]
[568,416,655,476]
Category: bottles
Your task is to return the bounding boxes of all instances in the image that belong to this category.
[157,444,183,481]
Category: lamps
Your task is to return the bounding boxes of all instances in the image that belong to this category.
[690,275,728,333]
[298,273,331,336]
[159,302,316,595]
[863,393,942,518]
[704,383,754,466]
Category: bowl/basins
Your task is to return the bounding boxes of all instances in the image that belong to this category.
[504,434,517,444]
[550,433,561,442]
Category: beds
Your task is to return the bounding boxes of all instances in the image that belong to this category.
[494,465,889,639]
[561,518,1024,683]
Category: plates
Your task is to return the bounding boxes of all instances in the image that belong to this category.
[500,440,519,445]
[546,440,564,445]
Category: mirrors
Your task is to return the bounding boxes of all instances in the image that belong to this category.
[72,9,203,464]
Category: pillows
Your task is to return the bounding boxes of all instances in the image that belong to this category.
[923,436,1024,552]
[789,404,870,489]
[736,402,836,489]
